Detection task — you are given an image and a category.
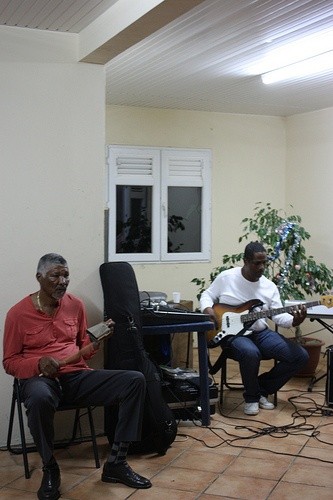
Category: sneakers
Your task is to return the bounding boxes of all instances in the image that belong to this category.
[259,395,274,410]
[244,402,259,414]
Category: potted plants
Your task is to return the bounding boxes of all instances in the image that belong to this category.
[191,201,333,377]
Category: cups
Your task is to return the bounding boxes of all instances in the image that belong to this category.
[171,292,181,304]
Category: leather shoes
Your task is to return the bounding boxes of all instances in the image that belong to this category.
[101,460,152,489]
[37,469,61,500]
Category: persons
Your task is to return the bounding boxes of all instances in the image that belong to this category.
[2,253,146,500]
[200,243,308,415]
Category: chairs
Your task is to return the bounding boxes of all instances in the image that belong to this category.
[7,377,101,479]
[220,324,278,406]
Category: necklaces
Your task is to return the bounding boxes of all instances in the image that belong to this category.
[36,290,42,311]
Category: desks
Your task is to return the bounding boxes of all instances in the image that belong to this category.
[140,321,215,427]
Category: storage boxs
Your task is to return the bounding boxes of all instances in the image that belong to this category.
[169,386,219,419]
[166,300,193,368]
[327,345,333,415]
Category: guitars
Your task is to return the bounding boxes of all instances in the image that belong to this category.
[206,292,333,349]
